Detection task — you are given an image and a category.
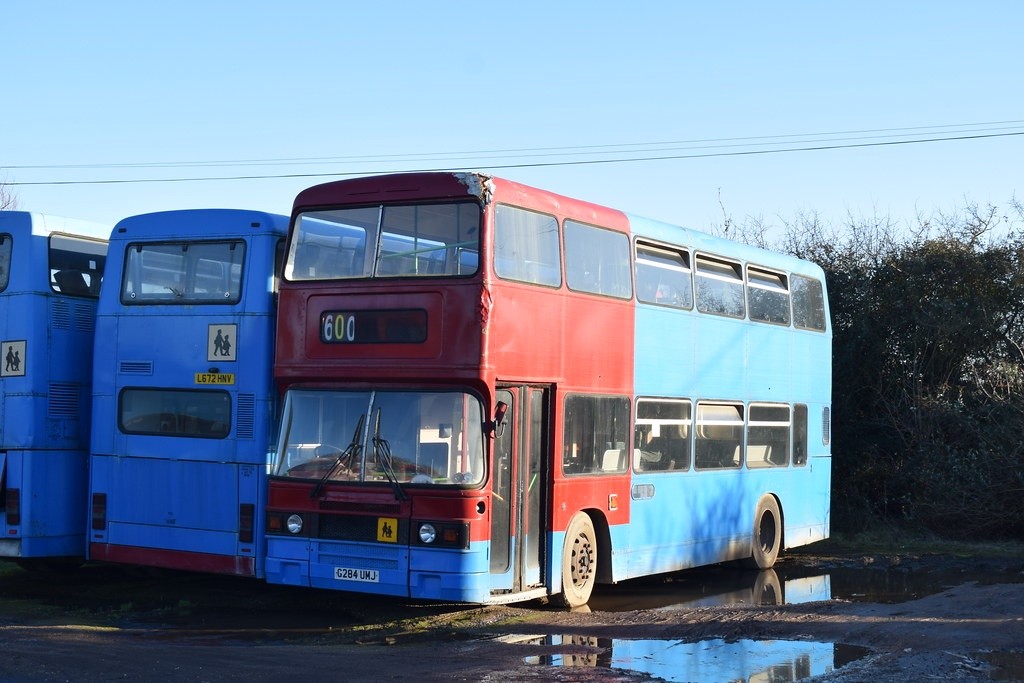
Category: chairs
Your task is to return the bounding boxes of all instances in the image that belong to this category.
[733,444,776,467]
[605,448,642,472]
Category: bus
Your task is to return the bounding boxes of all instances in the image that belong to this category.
[260,173,833,604]
[85,209,602,580]
[0,208,243,577]
